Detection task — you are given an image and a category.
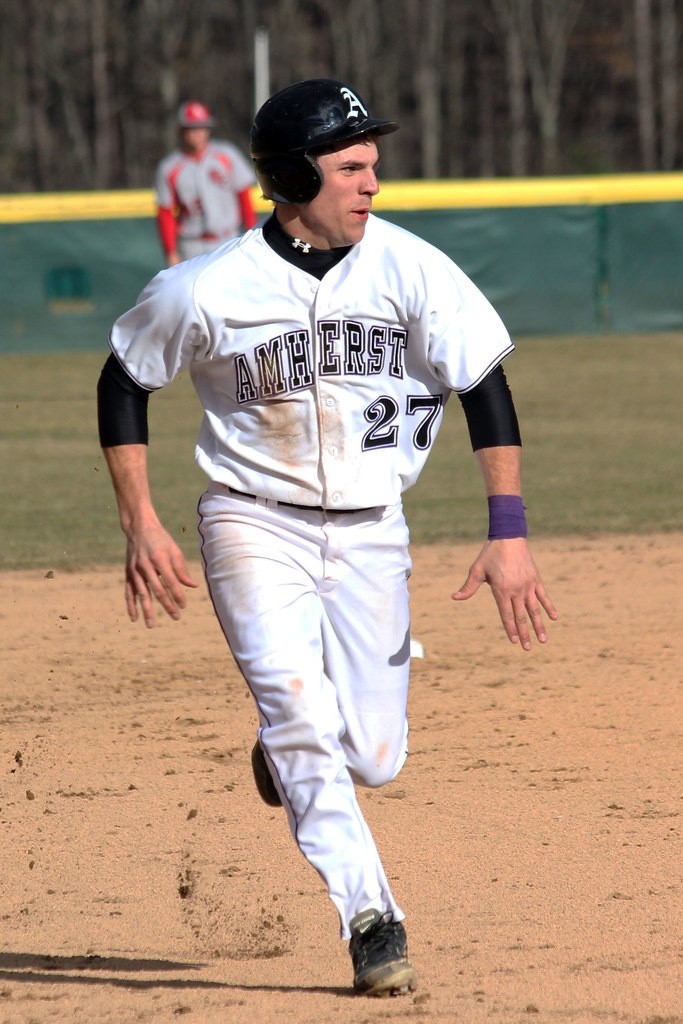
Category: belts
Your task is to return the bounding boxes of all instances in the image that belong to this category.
[229,480,376,513]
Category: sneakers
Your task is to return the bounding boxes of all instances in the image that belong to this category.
[251,738,285,808]
[348,908,418,997]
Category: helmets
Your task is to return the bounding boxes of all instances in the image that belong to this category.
[249,78,400,204]
[176,99,217,127]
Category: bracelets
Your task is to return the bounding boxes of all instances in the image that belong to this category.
[486,495,528,541]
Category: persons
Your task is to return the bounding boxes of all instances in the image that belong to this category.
[156,99,257,265]
[93,60,563,1002]
